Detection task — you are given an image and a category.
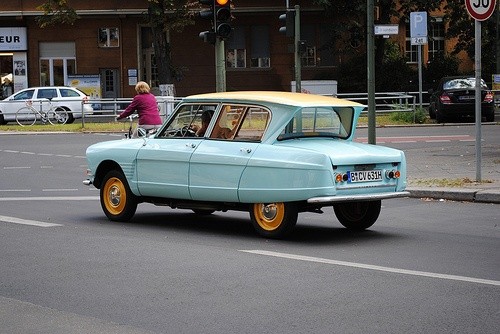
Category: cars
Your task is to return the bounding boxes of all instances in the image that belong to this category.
[82,91,410,238]
[0,86,94,125]
[428,76,495,123]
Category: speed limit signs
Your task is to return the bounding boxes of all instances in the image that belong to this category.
[465,0,496,21]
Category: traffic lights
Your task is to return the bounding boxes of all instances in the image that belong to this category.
[198,0,216,44]
[214,0,232,38]
[278,9,295,39]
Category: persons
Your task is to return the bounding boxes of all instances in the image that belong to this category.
[186,110,232,140]
[114,82,163,140]
[14,61,25,75]
[0,77,14,99]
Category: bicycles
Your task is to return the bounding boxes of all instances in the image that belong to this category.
[15,98,69,127]
[115,114,157,139]
